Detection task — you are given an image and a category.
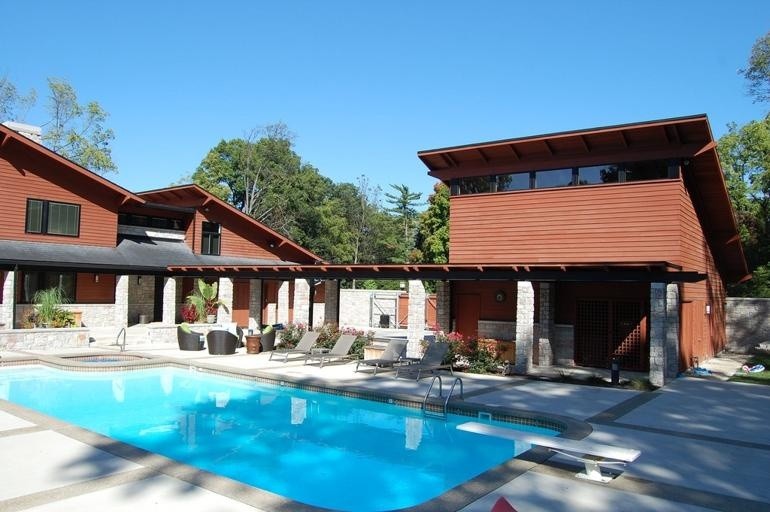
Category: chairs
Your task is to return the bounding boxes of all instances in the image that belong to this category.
[254,330,275,352]
[355,339,408,376]
[392,340,454,381]
[268,332,321,363]
[304,335,358,368]
[206,330,237,355]
[177,326,205,351]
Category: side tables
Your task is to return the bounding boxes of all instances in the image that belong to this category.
[399,358,421,376]
[310,348,330,363]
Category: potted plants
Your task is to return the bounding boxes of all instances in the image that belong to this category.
[33,288,70,329]
[186,279,226,323]
